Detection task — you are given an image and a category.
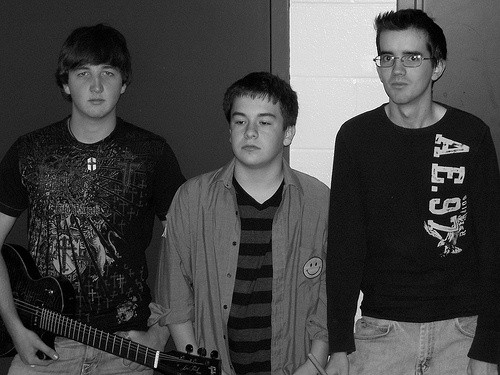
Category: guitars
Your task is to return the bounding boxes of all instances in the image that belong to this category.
[0,242,222,375]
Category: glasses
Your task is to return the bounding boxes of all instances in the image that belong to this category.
[373,53,435,68]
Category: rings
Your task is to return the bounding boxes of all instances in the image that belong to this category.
[30,364,36,368]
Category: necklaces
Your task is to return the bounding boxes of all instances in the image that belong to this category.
[69,118,77,140]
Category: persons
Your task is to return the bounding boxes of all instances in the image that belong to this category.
[147,71,331,375]
[0,21,189,368]
[323,6,500,375]
